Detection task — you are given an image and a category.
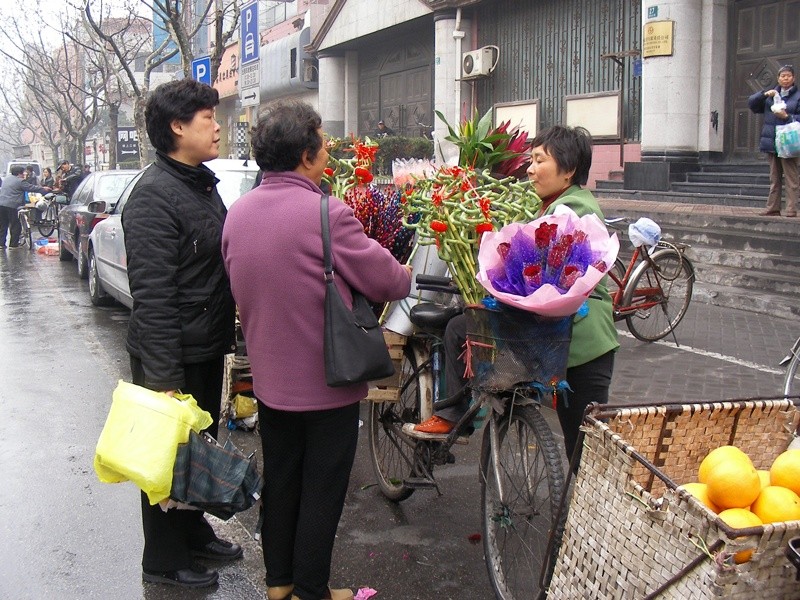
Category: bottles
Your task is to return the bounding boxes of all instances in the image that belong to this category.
[773,93,781,104]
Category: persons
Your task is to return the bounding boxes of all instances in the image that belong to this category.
[747,66,800,217]
[400,126,618,470]
[0,159,93,249]
[236,103,412,600]
[120,80,229,587]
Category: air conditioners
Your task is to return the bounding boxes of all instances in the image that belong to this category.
[462,49,492,78]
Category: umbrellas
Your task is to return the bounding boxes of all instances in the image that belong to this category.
[168,417,260,523]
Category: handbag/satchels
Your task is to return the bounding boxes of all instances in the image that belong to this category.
[775,116,800,158]
[95,377,215,506]
[318,196,395,386]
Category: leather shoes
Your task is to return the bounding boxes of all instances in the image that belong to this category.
[141,558,220,587]
[190,537,244,560]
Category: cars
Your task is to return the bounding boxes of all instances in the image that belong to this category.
[55,169,143,279]
[86,159,261,310]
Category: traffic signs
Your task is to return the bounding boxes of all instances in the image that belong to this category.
[117,126,140,163]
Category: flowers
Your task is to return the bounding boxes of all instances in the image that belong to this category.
[496,221,606,294]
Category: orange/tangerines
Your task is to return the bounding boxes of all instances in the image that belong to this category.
[678,446,800,564]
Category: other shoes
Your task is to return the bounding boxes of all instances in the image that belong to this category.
[786,210,797,217]
[268,584,353,600]
[0,244,7,249]
[758,209,780,216]
[10,244,22,249]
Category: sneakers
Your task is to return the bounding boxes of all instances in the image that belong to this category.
[402,415,470,445]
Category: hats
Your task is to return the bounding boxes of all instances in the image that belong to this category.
[379,120,384,124]
[55,158,66,171]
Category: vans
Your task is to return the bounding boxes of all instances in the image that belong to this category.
[5,159,42,183]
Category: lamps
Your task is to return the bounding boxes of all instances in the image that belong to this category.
[292,18,304,28]
[199,41,216,54]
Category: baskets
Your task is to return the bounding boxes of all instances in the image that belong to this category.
[462,302,575,393]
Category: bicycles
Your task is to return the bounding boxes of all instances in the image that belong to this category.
[37,193,59,237]
[368,273,565,600]
[17,206,32,250]
[604,216,696,347]
